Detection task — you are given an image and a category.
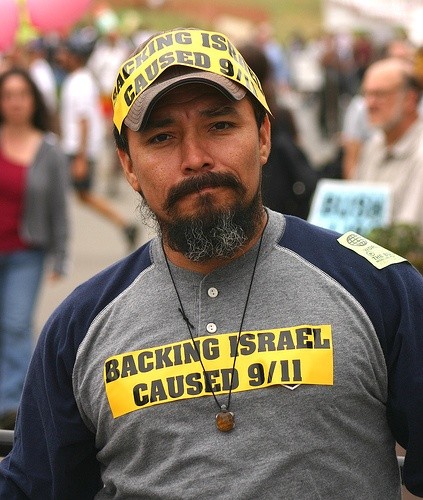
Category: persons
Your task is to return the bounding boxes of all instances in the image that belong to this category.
[0,24,423,500]
[0,66,70,420]
[347,58,423,229]
[0,9,423,284]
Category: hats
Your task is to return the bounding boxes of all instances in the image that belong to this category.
[122,27,248,132]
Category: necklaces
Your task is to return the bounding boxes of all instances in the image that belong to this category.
[160,203,271,434]
